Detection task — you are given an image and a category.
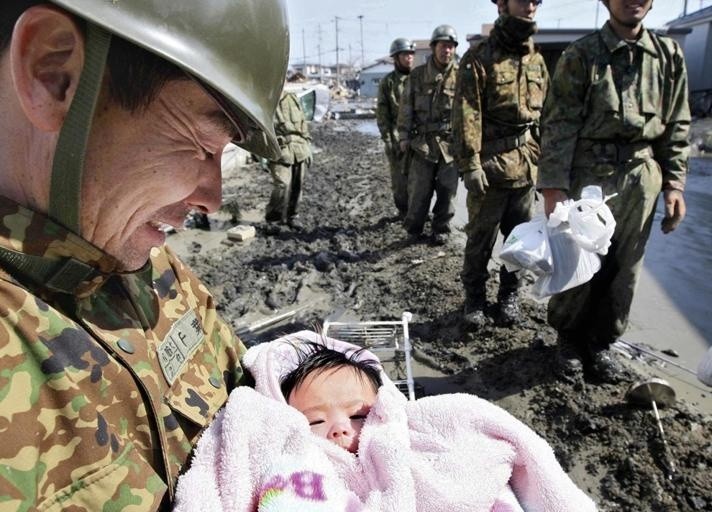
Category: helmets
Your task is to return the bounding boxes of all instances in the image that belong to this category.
[53,0,289,162]
[429,25,458,47]
[390,39,417,57]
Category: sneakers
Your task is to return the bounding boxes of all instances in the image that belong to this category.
[267,220,281,233]
[594,350,623,381]
[497,292,521,325]
[287,219,303,228]
[561,358,584,381]
[464,299,484,329]
[432,233,449,243]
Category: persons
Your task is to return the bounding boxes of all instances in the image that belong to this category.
[280,336,383,460]
[451,0,551,327]
[395,24,459,246]
[536,0,696,377]
[261,92,315,235]
[1,2,290,512]
[376,38,418,222]
